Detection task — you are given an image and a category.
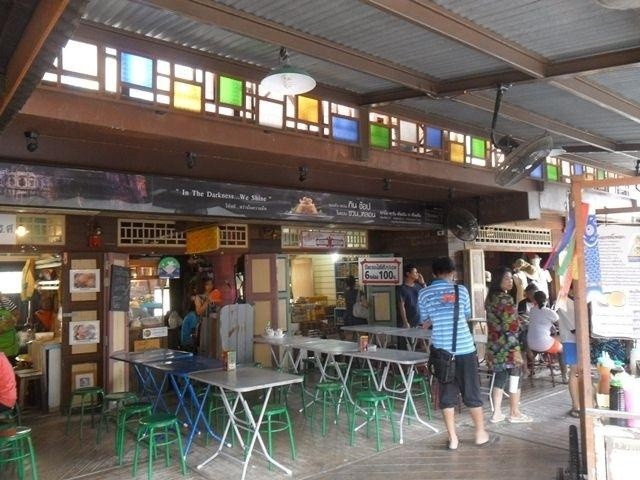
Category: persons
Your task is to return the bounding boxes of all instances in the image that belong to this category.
[194,276,221,317]
[0,350,17,413]
[180,302,200,350]
[396,254,598,450]
[342,275,367,325]
[0,291,22,362]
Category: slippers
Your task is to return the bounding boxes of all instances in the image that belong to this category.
[473,430,498,447]
[489,413,505,423]
[507,414,535,423]
[448,435,462,449]
[569,408,581,418]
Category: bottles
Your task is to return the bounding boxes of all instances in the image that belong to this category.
[595,361,626,423]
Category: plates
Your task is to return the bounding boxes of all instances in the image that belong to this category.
[262,333,285,339]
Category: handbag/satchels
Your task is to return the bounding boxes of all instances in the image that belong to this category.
[428,342,456,384]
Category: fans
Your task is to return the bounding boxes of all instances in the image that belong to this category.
[441,185,480,243]
[483,82,555,190]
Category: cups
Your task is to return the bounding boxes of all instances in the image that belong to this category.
[267,329,282,336]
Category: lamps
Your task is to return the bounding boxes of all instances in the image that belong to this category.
[25,129,393,193]
[15,217,29,237]
[257,46,317,96]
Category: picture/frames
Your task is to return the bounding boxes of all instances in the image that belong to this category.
[69,268,102,293]
[71,368,97,390]
[67,319,99,345]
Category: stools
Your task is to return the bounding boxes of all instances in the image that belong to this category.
[254,334,431,452]
[530,347,567,386]
[0,388,296,479]
[14,367,43,404]
